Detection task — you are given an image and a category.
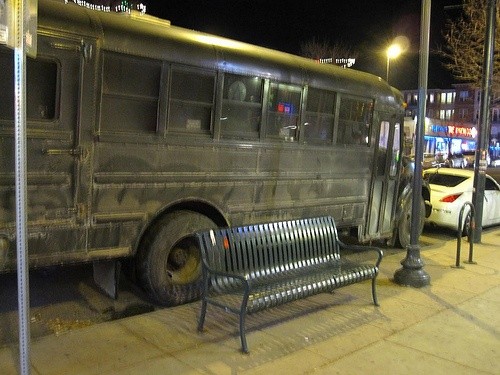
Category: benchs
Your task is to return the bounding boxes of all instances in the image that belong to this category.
[194,216,384,354]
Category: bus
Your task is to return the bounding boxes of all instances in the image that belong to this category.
[0,0,432,308]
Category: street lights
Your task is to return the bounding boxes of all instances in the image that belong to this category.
[385,42,401,82]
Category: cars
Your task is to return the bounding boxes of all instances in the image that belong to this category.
[420,168,500,236]
[422,144,493,169]
